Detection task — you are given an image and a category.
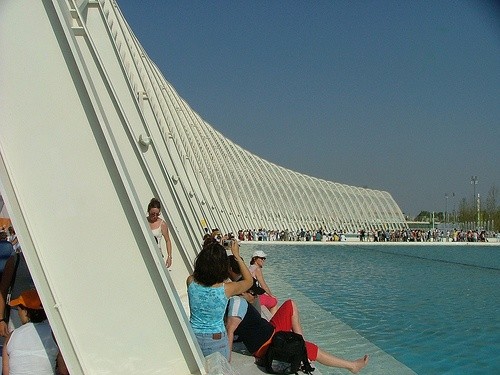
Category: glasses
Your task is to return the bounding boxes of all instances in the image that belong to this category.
[258,257,266,260]
[149,211,161,216]
[249,292,258,297]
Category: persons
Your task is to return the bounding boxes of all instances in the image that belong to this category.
[0,252,37,337]
[247,250,278,315]
[145,197,173,268]
[2,288,70,375]
[209,226,491,252]
[0,225,22,251]
[224,276,369,375]
[185,237,253,362]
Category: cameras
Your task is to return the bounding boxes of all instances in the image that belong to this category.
[223,240,233,249]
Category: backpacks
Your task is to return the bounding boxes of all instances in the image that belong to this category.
[254,331,315,375]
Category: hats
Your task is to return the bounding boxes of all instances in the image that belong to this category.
[8,291,42,309]
[237,276,266,295]
[252,250,267,257]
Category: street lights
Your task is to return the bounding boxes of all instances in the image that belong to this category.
[444,192,449,223]
[452,192,456,224]
